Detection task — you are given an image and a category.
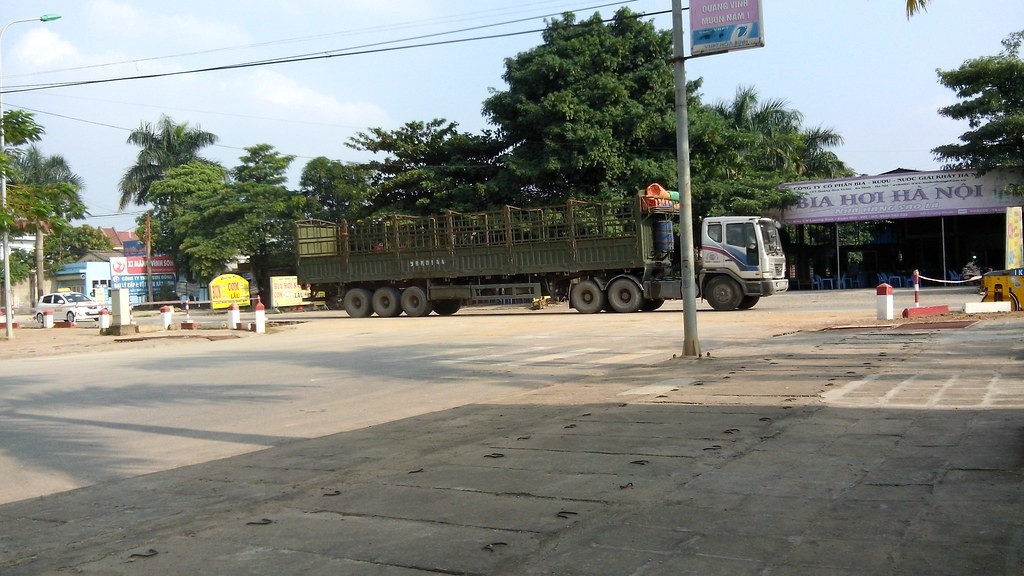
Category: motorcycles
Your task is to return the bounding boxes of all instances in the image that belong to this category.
[961,255,983,286]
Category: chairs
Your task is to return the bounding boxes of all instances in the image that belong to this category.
[808,269,961,290]
[732,230,744,246]
[717,232,731,243]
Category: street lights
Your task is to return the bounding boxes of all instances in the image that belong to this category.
[0,13,63,338]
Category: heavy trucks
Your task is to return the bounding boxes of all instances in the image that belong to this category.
[297,186,789,319]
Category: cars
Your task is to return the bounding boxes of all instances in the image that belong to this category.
[35,293,107,323]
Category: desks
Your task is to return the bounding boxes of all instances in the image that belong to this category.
[532,299,548,309]
